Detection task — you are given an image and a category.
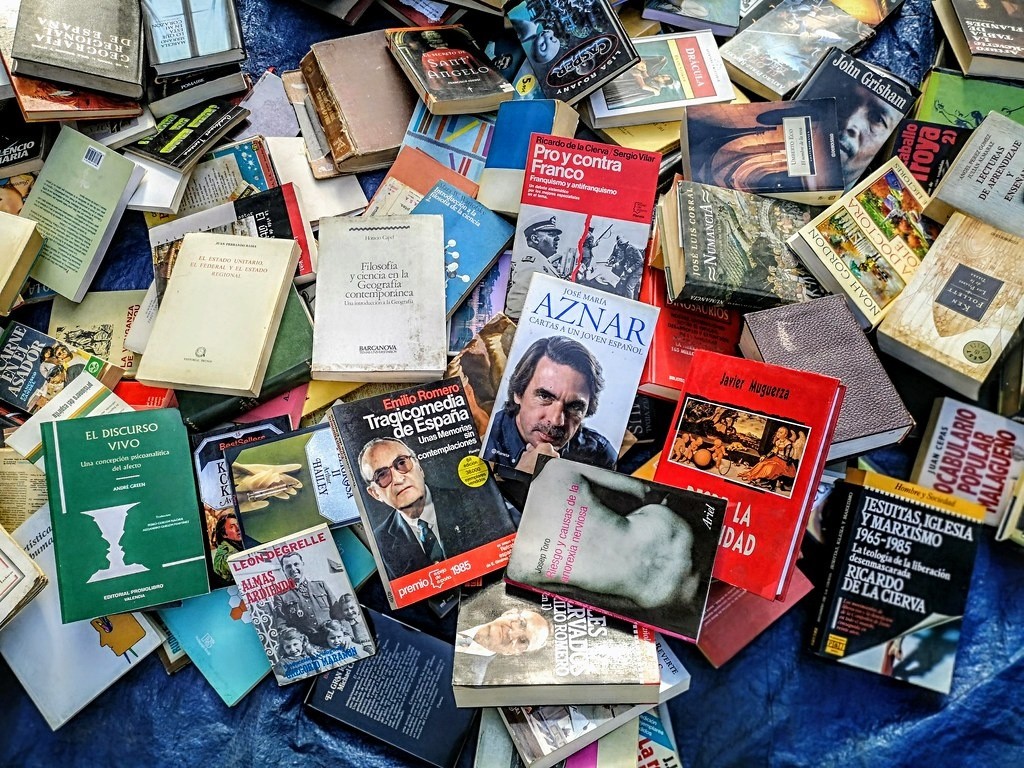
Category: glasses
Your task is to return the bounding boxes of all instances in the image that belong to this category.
[513,611,529,654]
[368,454,413,488]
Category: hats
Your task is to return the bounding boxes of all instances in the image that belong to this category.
[522,212,562,237]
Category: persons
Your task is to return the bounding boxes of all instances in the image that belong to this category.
[835,82,904,185]
[357,437,505,581]
[274,551,354,661]
[315,619,369,673]
[483,335,617,475]
[613,238,644,300]
[339,592,375,656]
[505,212,563,317]
[607,234,625,264]
[213,513,245,581]
[578,227,600,268]
[273,627,322,687]
[452,602,549,686]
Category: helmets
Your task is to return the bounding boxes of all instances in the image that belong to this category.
[616,235,624,240]
[590,224,595,228]
[617,238,629,247]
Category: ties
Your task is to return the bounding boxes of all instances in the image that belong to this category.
[417,520,445,563]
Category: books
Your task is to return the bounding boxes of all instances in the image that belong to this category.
[0,0,1024,768]
